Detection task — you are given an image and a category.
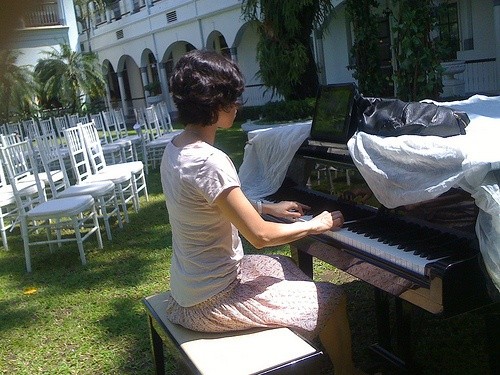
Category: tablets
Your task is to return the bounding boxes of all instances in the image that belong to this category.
[309,83,356,143]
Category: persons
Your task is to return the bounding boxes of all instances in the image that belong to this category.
[160,52,383,375]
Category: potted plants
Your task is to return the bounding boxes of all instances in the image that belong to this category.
[435,30,466,98]
[142,82,162,106]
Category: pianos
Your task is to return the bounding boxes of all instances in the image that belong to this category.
[248,96,500,374]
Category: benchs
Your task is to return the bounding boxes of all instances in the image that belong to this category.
[142,287,337,375]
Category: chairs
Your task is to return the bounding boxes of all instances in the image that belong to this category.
[0,100,185,273]
